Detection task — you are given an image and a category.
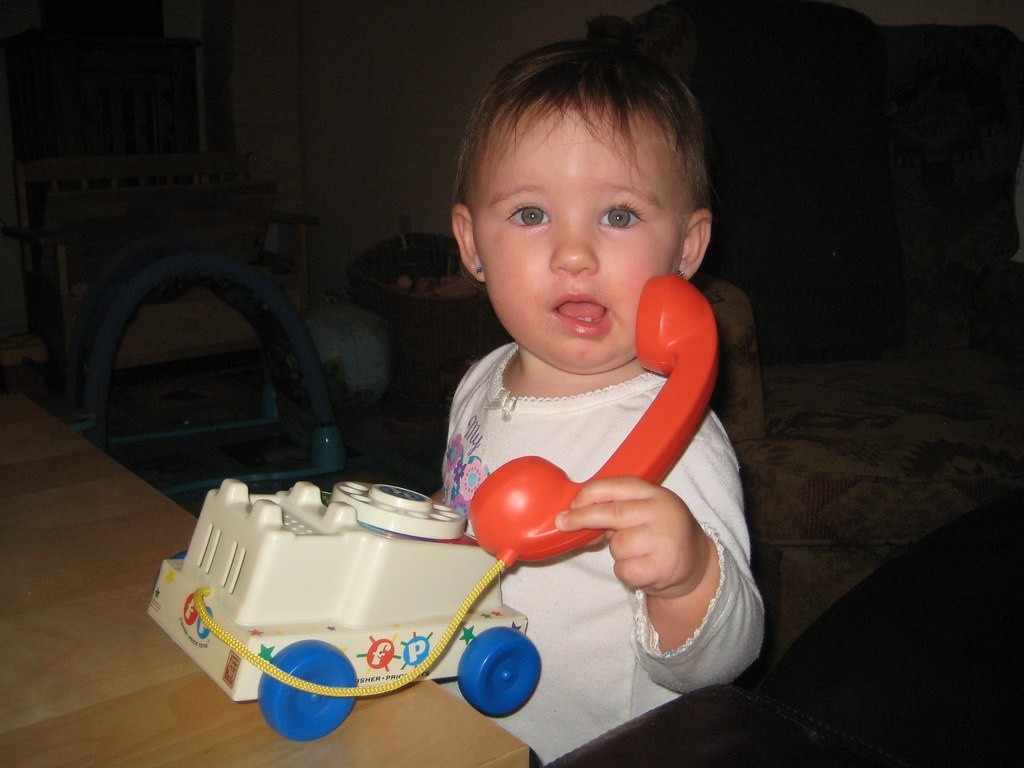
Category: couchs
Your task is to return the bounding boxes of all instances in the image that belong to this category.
[584,2,1024,661]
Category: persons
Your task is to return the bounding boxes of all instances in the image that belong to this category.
[422,3,768,768]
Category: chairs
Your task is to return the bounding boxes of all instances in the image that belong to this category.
[0,155,320,429]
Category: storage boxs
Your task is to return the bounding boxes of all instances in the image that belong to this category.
[341,221,519,414]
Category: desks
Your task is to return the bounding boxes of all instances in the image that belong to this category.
[0,210,320,262]
[1,376,529,768]
